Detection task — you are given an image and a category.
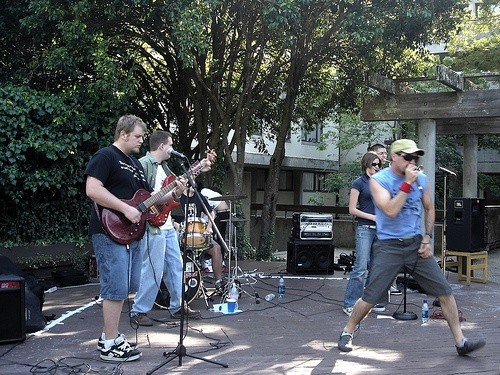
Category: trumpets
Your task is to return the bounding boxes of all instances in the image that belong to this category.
[383,159,425,173]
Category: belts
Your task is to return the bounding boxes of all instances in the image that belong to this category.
[363,225,376,229]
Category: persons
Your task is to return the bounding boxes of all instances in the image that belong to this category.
[338,139,486,357]
[129,131,211,327]
[172,182,233,296]
[83,114,187,362]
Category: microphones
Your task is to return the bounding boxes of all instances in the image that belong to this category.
[415,176,422,190]
[167,147,186,158]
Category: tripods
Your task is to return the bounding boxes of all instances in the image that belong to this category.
[218,199,260,304]
[144,156,229,375]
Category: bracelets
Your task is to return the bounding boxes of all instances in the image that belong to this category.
[399,182,412,194]
[426,232,433,239]
[171,188,181,200]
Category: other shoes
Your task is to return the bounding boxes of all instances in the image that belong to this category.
[342,306,354,316]
[455,340,486,357]
[338,330,353,351]
[389,286,402,295]
[371,303,385,312]
[130,315,153,326]
[170,302,201,319]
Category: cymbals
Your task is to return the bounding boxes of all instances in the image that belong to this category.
[221,218,248,222]
[209,195,248,202]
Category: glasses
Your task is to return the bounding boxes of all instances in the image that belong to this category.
[396,153,420,161]
[372,163,380,166]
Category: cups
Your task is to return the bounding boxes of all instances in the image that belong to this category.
[227,300,236,313]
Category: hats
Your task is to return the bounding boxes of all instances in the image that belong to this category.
[390,139,426,156]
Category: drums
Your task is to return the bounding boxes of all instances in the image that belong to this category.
[182,217,206,245]
[171,220,182,245]
[186,232,213,251]
[153,252,201,309]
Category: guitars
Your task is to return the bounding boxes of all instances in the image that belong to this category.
[101,160,202,245]
[150,149,217,227]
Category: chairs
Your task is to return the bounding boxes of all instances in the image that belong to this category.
[442,225,488,285]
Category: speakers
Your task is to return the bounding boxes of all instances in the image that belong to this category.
[446,197,486,252]
[287,240,335,275]
[0,274,26,343]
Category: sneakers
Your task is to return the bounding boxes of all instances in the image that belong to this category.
[97,332,138,350]
[100,337,143,362]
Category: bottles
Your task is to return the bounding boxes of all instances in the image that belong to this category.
[265,293,275,302]
[278,275,286,299]
[230,283,238,301]
[421,299,430,323]
